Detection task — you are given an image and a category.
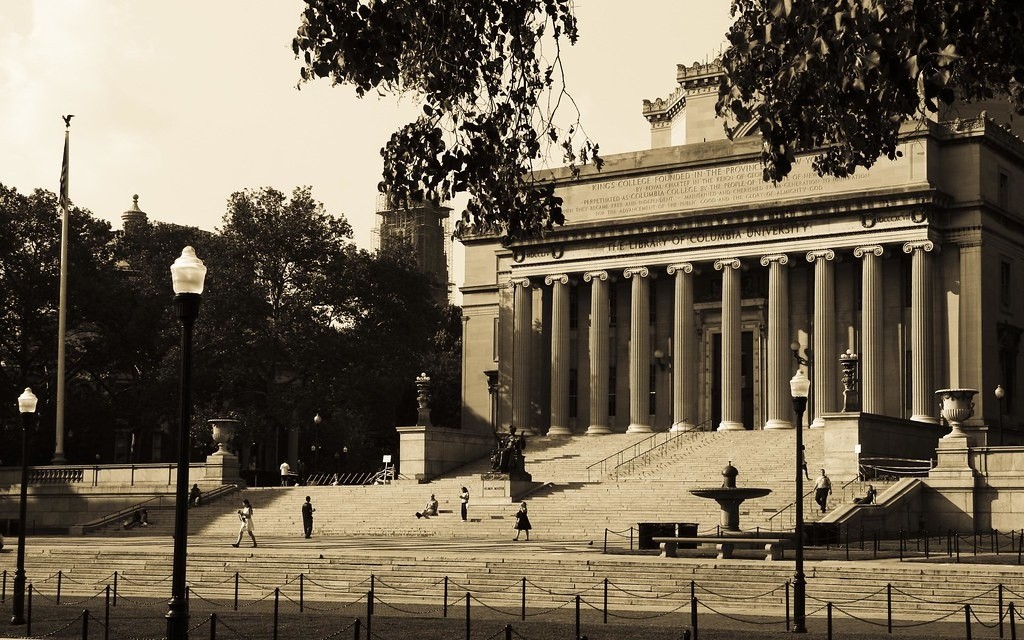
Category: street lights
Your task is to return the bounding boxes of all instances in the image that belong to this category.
[790,368,810,634]
[311,413,322,472]
[164,246,207,640]
[995,385,1005,446]
[11,388,38,625]
[342,446,347,474]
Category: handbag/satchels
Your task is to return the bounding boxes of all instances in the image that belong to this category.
[514,521,519,529]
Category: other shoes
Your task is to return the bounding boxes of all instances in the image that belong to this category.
[252,544,257,548]
[513,539,518,541]
[233,544,240,548]
[305,536,311,539]
[807,478,813,480]
[416,512,422,519]
[462,519,467,522]
[525,539,530,541]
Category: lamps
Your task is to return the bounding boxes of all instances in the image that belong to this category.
[654,350,667,372]
[790,341,808,366]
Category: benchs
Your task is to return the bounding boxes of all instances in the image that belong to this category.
[652,537,792,562]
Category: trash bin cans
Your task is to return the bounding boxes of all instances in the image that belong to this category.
[660,522,677,543]
[637,522,661,549]
[677,522,699,549]
[822,522,841,545]
[803,521,824,546]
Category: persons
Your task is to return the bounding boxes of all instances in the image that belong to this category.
[302,496,316,539]
[812,469,832,513]
[490,425,526,473]
[123,509,148,530]
[297,459,306,474]
[188,484,202,510]
[853,485,877,504]
[231,499,257,548]
[460,486,469,522]
[280,461,290,486]
[416,494,438,519]
[802,445,813,481]
[513,502,532,541]
